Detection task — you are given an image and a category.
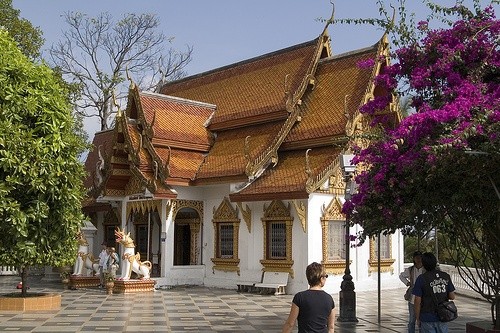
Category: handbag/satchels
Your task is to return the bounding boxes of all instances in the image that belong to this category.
[404,287,412,300]
[436,301,457,322]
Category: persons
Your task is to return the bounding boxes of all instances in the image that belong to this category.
[282,262,336,333]
[399,251,456,333]
[97,241,119,288]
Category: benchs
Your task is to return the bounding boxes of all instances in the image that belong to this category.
[254,271,290,296]
[236,270,264,294]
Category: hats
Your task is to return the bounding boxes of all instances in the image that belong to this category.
[414,251,422,257]
[101,242,108,245]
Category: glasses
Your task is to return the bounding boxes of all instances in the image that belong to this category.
[321,275,328,278]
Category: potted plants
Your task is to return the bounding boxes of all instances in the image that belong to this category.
[104,275,115,295]
[61,271,70,290]
